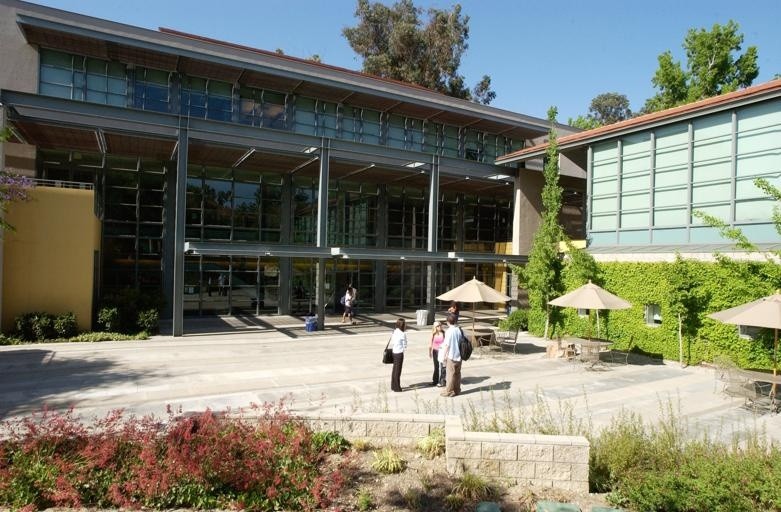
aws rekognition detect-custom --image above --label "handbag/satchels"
[382,349,394,364]
[340,295,345,305]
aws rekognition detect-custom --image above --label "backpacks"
[458,326,473,362]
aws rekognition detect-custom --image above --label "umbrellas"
[435,280,513,332]
[704,295,781,372]
[546,283,635,336]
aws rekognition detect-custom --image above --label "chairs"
[547,331,635,371]
[458,325,520,359]
[710,358,781,416]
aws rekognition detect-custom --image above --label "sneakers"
[439,390,460,397]
[428,381,444,387]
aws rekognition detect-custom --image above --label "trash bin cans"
[306,318,317,332]
[416,310,428,325]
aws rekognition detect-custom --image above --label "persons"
[208,274,213,297]
[428,321,446,388]
[217,273,224,297]
[342,280,358,326]
[391,319,409,392]
[447,302,460,324]
[441,314,466,397]
[223,275,230,297]
